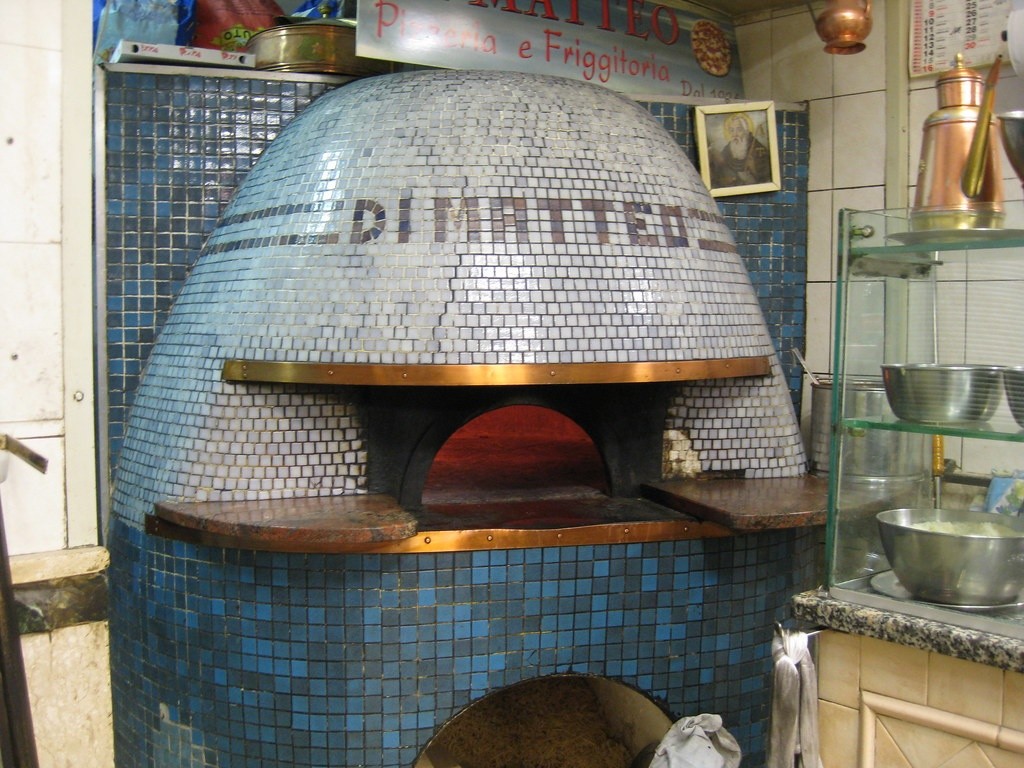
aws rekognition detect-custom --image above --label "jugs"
[910,53,1005,232]
[807,0,873,55]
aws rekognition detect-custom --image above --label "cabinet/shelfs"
[824,210,1024,639]
[813,629,1024,768]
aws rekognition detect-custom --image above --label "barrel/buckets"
[809,378,928,486]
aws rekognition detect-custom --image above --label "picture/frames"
[693,100,782,198]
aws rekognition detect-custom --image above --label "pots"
[245,7,396,76]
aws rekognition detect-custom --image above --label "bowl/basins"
[876,509,1024,615]
[880,363,1008,424]
[1002,365,1024,428]
[996,110,1024,189]
